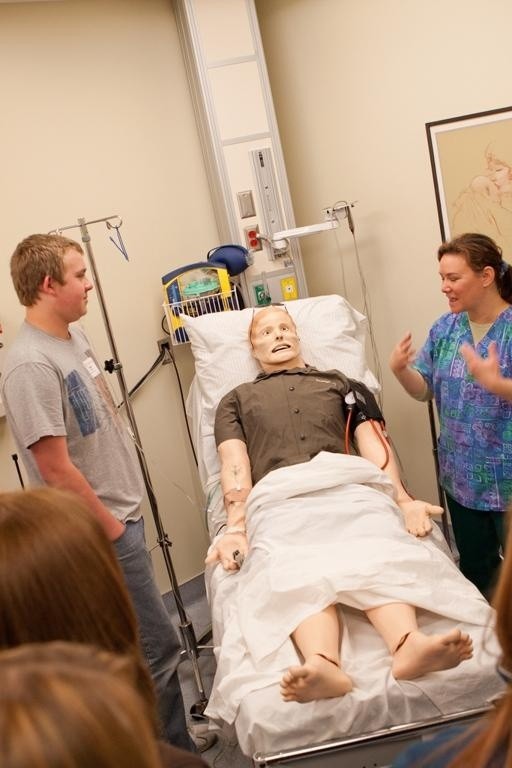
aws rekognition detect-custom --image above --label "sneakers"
[185,723,220,754]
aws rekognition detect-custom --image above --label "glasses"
[252,303,291,321]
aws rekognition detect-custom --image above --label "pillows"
[178,294,382,426]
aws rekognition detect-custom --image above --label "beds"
[183,370,511,767]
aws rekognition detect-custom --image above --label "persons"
[484,134,512,211]
[0,638,160,767]
[0,232,219,756]
[469,173,502,207]
[0,483,156,710]
[392,498,511,768]
[203,303,474,704]
[389,230,512,598]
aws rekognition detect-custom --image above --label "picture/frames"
[424,105,512,265]
